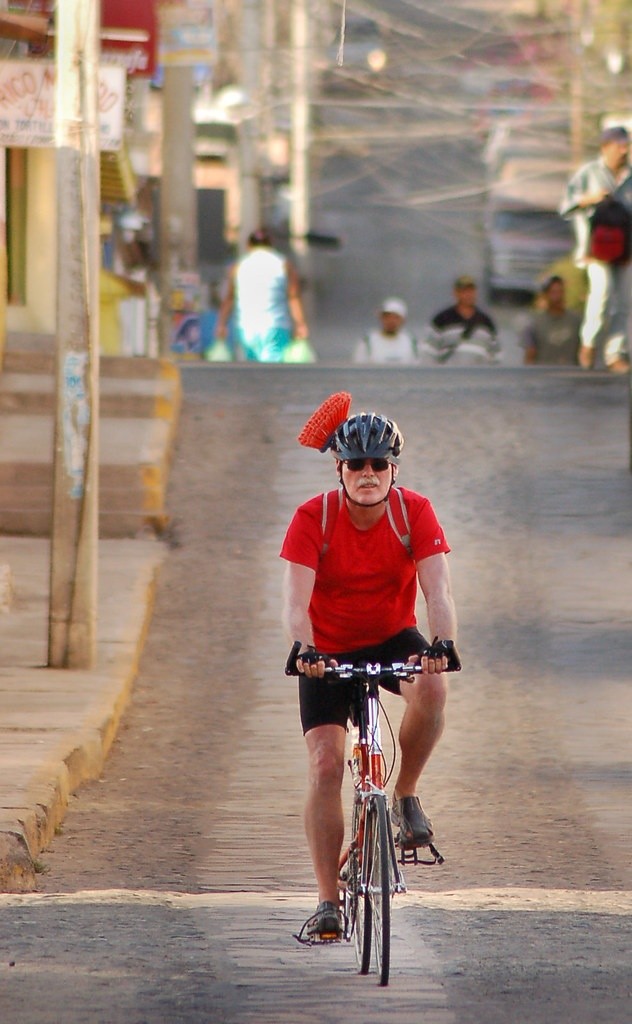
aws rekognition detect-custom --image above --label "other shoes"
[578,344,595,371]
[606,356,632,375]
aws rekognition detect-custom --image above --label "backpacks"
[588,197,632,266]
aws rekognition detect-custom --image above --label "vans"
[483,179,582,297]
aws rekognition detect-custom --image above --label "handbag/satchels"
[204,339,235,365]
[279,341,318,365]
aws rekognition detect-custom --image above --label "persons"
[416,273,503,367]
[347,295,419,365]
[215,227,310,362]
[520,275,607,371]
[558,127,632,374]
[280,412,462,935]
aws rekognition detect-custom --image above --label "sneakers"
[316,901,343,934]
[391,793,435,848]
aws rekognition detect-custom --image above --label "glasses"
[342,458,394,472]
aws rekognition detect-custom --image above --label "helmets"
[327,413,405,461]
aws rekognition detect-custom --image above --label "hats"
[382,296,409,319]
[455,276,476,290]
[598,126,626,146]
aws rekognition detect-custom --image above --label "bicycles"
[282,640,462,985]
